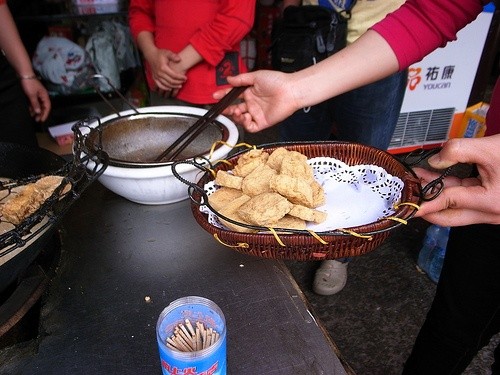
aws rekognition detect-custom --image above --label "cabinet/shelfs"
[33,68,150,156]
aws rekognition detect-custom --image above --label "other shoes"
[313,259,349,295]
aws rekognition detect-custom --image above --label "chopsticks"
[152,85,250,163]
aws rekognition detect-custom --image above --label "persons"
[129,0,255,144]
[0,0,50,159]
[212,0,500,375]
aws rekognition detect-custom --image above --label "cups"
[156,295,229,375]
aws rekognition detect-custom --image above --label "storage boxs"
[48,119,88,146]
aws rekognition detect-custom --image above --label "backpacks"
[270,0,356,112]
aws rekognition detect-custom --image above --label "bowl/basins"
[72,105,239,206]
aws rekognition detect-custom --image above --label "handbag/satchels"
[32,18,143,94]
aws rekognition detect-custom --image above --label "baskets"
[170,140,452,261]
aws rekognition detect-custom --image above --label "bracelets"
[19,75,38,80]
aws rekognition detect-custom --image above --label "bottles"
[417,222,451,283]
